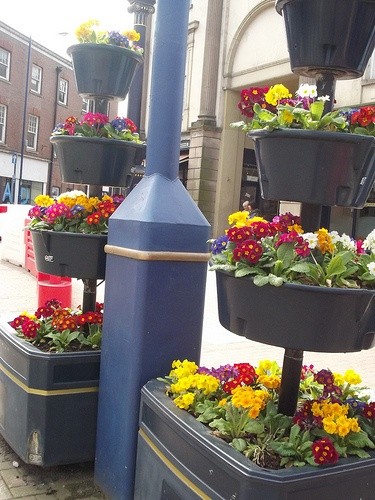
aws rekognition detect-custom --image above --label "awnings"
[131,151,189,176]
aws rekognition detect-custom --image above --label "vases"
[248,130,375,209]
[134,376,375,500]
[0,317,102,467]
[30,230,108,280]
[274,0,375,80]
[50,136,146,187]
[215,270,375,352]
[66,44,143,100]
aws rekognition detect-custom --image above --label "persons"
[242,201,251,212]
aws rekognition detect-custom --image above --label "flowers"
[75,19,144,55]
[158,356,375,469]
[206,210,375,289]
[51,112,141,140]
[23,190,127,235]
[229,83,375,138]
[8,298,107,353]
